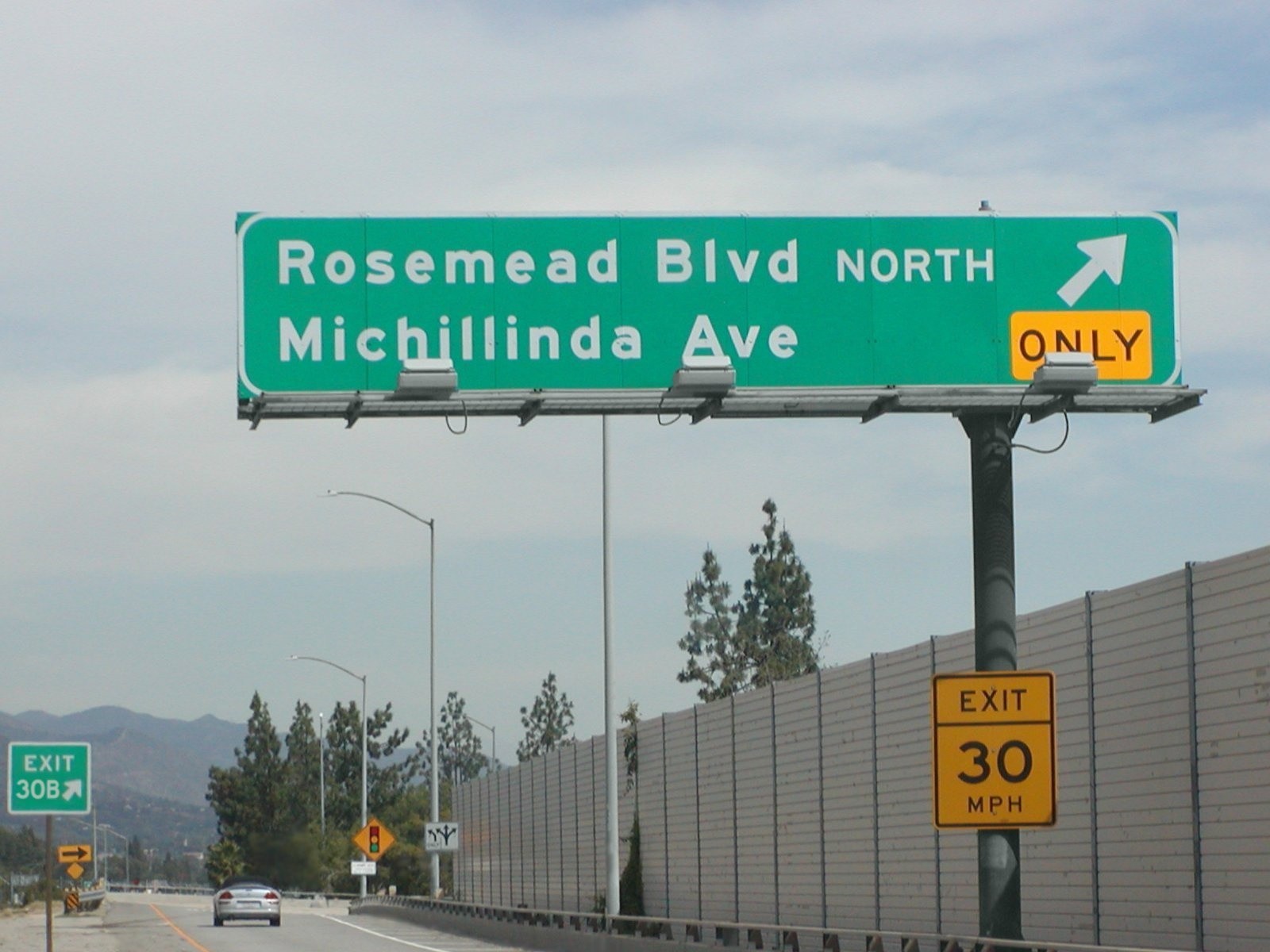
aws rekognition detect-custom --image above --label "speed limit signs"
[928,670,1058,829]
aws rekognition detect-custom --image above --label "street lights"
[449,711,496,772]
[286,654,369,897]
[97,822,112,891]
[319,490,442,900]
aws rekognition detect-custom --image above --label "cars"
[210,881,284,928]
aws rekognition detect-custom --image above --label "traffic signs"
[232,207,1187,408]
[7,740,91,815]
